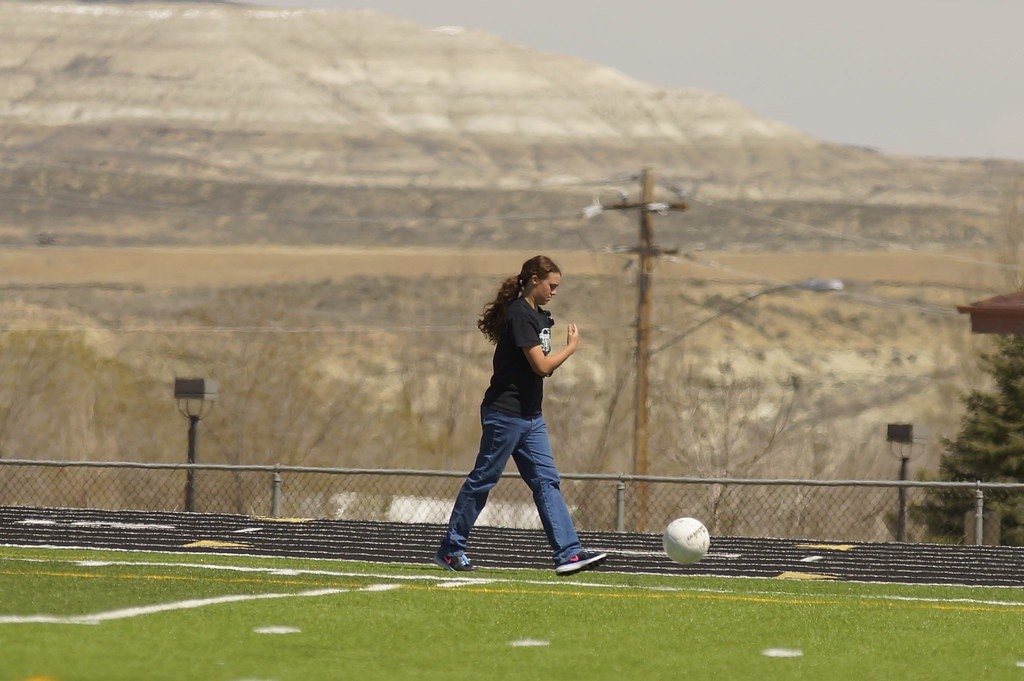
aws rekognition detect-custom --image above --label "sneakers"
[555,551,608,575]
[433,554,477,572]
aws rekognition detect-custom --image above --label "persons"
[434,255,608,575]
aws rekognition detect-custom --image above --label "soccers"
[662,517,711,564]
